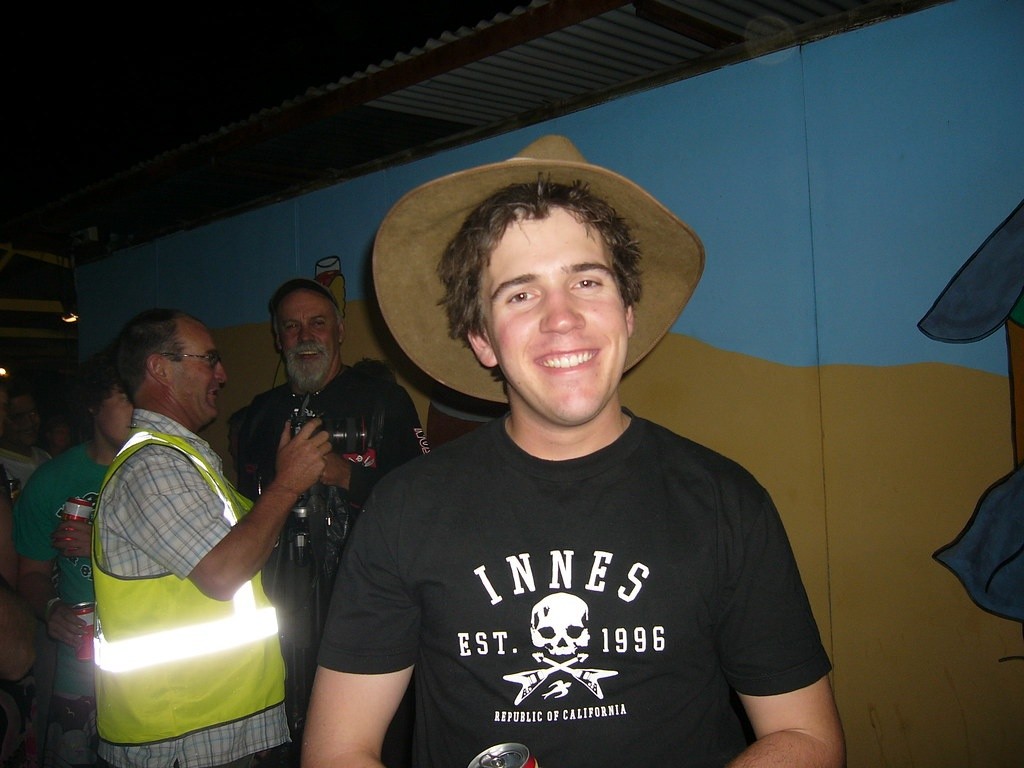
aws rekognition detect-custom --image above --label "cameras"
[290,391,369,463]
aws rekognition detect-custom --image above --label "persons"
[91,313,331,768]
[304,136,839,768]
[0,368,135,768]
[226,279,433,768]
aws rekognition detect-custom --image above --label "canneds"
[70,601,96,661]
[467,742,541,768]
[53,498,92,550]
[8,478,21,506]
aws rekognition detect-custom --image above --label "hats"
[372,135,707,403]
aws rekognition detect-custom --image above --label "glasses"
[158,352,221,369]
